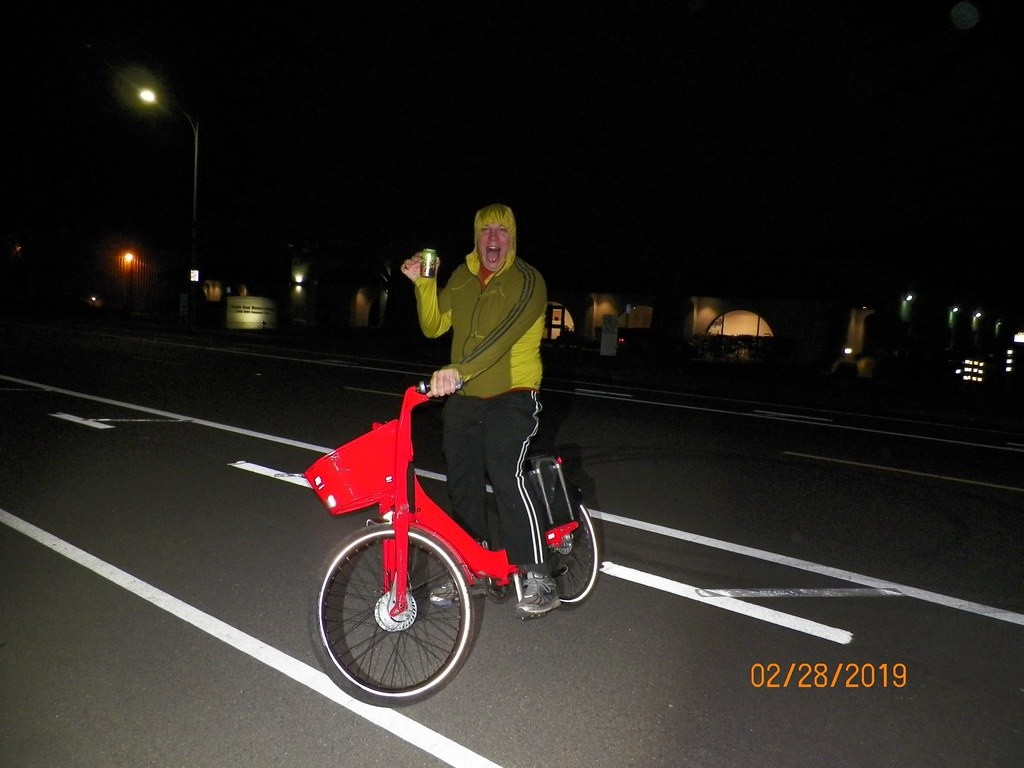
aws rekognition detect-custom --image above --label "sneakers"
[427,579,489,606]
[513,577,565,619]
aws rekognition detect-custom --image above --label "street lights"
[134,86,202,209]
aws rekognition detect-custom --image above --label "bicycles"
[303,376,602,709]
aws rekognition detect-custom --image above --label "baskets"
[305,418,400,515]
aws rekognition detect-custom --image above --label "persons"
[400,203,562,618]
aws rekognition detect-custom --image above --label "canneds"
[420,248,435,278]
